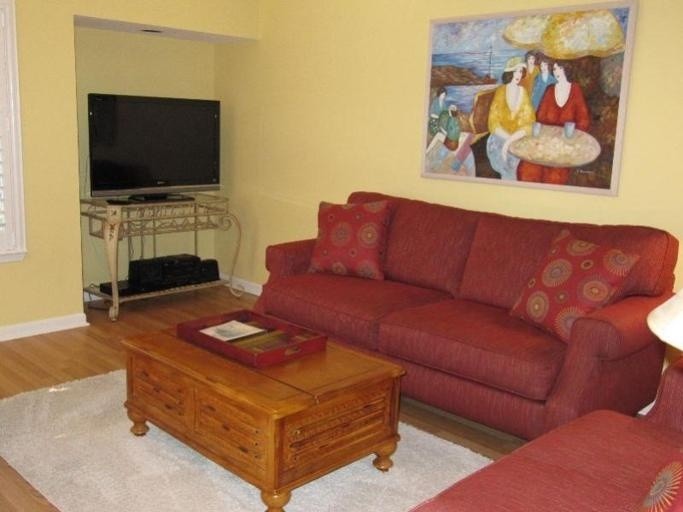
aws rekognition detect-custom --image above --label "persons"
[483,50,591,188]
[428,85,446,136]
[424,103,460,157]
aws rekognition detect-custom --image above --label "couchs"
[409,354,683,512]
[254,190,679,442]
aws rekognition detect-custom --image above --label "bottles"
[444,109,462,151]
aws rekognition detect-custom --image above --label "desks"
[639,289,683,417]
[79,192,244,321]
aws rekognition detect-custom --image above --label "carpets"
[0,365,493,512]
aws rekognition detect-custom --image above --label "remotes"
[106,199,131,205]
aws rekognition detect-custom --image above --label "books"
[198,320,264,342]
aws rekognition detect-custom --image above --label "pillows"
[509,229,640,345]
[308,199,398,282]
[633,458,683,512]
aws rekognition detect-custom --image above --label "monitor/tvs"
[86,92,221,203]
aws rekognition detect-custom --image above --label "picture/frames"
[418,0,638,199]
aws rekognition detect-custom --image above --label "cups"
[532,121,542,138]
[564,121,576,138]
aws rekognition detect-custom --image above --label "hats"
[502,55,527,74]
